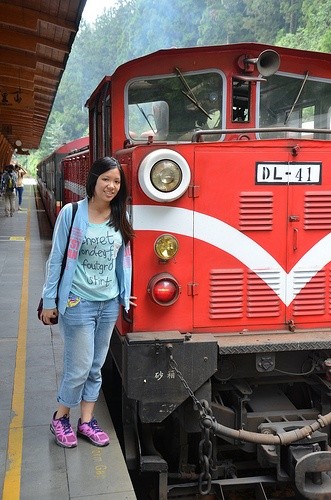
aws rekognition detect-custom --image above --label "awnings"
[0,0,87,150]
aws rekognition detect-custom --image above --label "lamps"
[15,137,22,147]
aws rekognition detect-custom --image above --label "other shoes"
[10,212,14,217]
[18,207,23,211]
[5,212,9,217]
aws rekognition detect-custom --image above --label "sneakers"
[50,410,78,448]
[76,416,109,447]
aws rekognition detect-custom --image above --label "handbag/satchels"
[37,298,59,325]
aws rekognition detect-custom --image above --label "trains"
[35,41,331,496]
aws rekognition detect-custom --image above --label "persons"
[40,156,137,449]
[0,163,27,217]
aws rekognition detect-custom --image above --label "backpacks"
[2,172,15,192]
